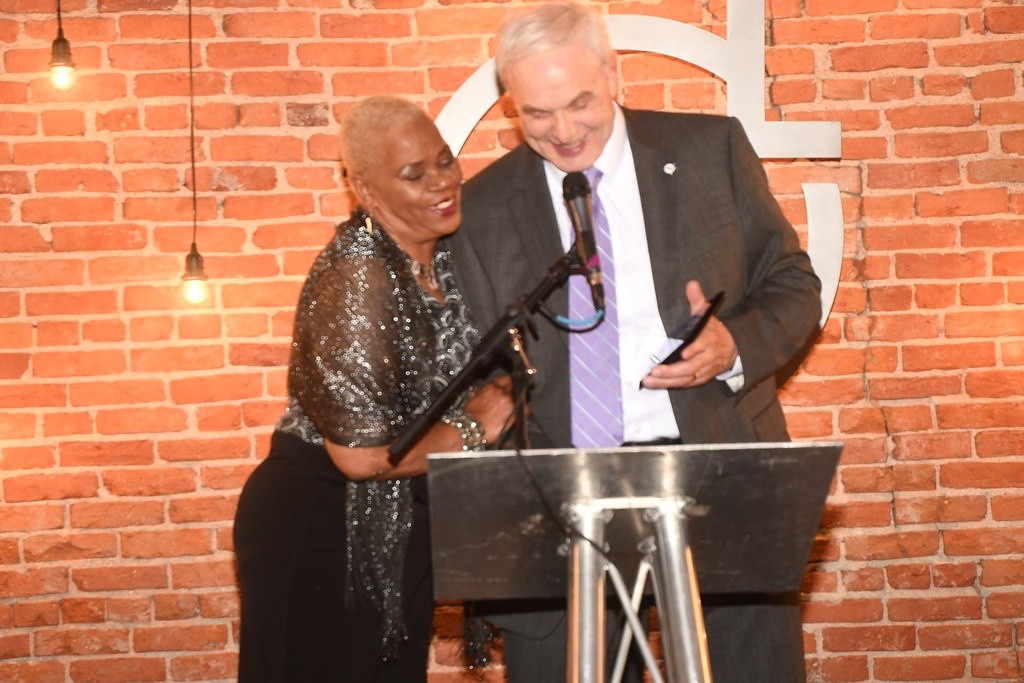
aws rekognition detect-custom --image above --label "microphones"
[564,172,606,311]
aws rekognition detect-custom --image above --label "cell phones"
[638,292,724,389]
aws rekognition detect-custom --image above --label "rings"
[693,374,697,381]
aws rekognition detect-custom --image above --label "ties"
[568,167,623,449]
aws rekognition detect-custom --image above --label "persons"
[233,95,529,683]
[437,4,822,683]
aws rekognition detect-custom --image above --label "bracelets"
[441,409,486,450]
